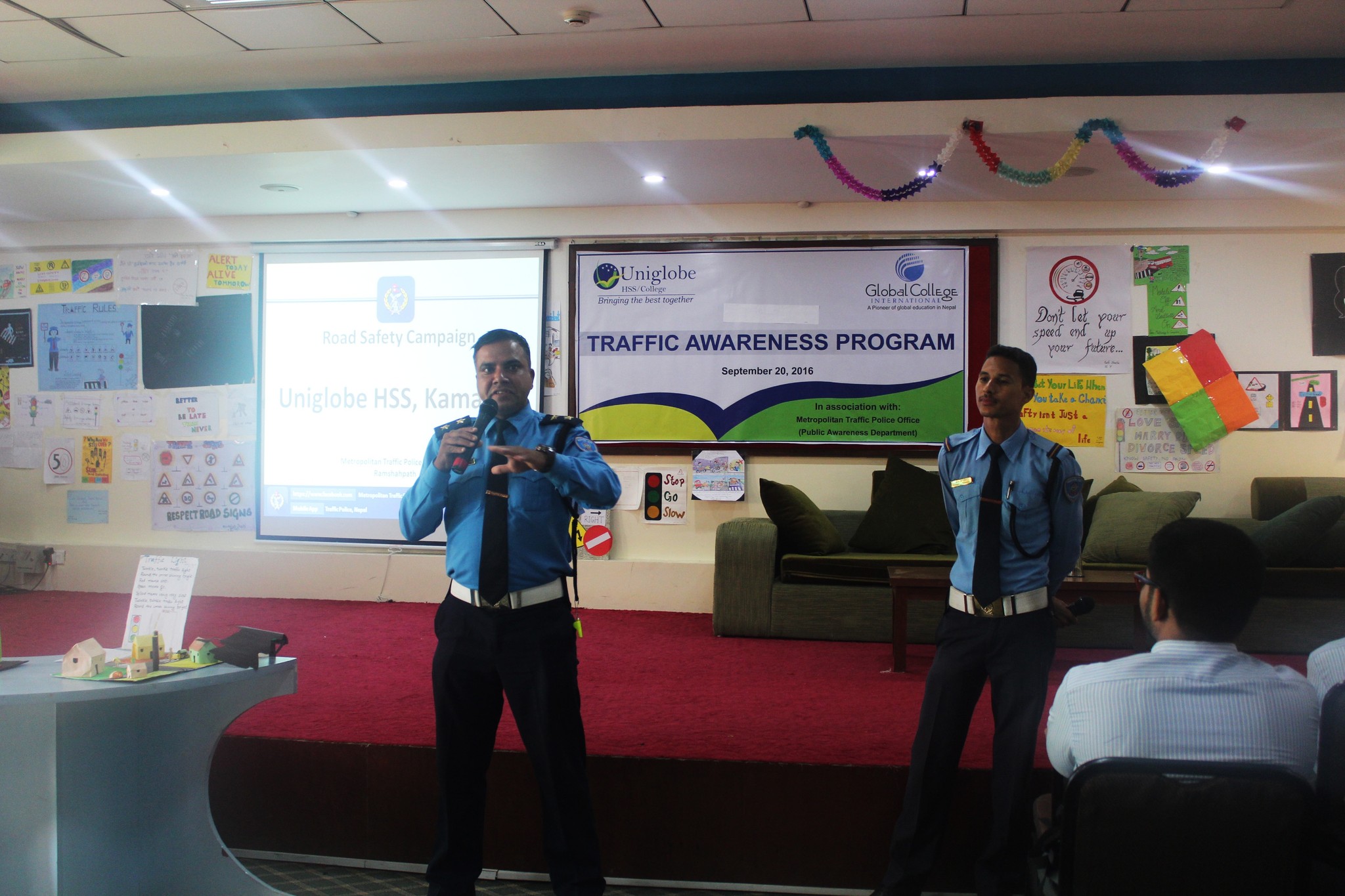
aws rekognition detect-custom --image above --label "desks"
[0,655,297,896]
[886,565,1148,672]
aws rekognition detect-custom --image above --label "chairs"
[1060,757,1315,896]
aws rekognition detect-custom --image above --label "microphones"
[451,398,498,475]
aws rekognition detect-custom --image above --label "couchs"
[712,478,1345,653]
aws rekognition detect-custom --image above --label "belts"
[948,586,1048,618]
[450,578,564,609]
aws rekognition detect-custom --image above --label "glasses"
[1134,570,1168,600]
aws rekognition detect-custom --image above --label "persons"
[1306,636,1345,776]
[869,344,1086,896]
[1045,518,1321,838]
[398,329,621,896]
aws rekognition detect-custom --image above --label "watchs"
[535,443,555,474]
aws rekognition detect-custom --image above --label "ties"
[477,419,511,607]
[972,443,1006,608]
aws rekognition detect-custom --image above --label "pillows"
[1080,475,1144,549]
[760,477,847,556]
[848,452,958,555]
[1081,491,1202,564]
[1249,493,1345,567]
